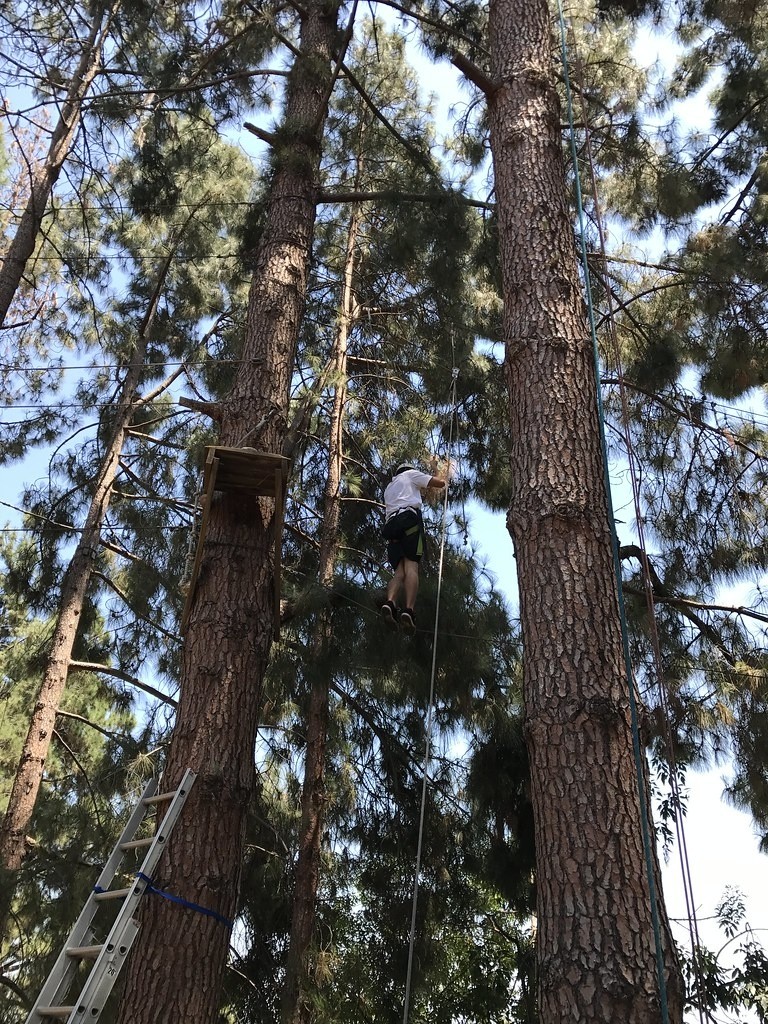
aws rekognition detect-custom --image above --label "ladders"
[24,767,198,1024]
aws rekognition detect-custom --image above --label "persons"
[381,460,446,637]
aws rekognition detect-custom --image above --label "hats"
[395,463,414,476]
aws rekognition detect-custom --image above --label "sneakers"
[401,609,416,636]
[381,602,399,632]
